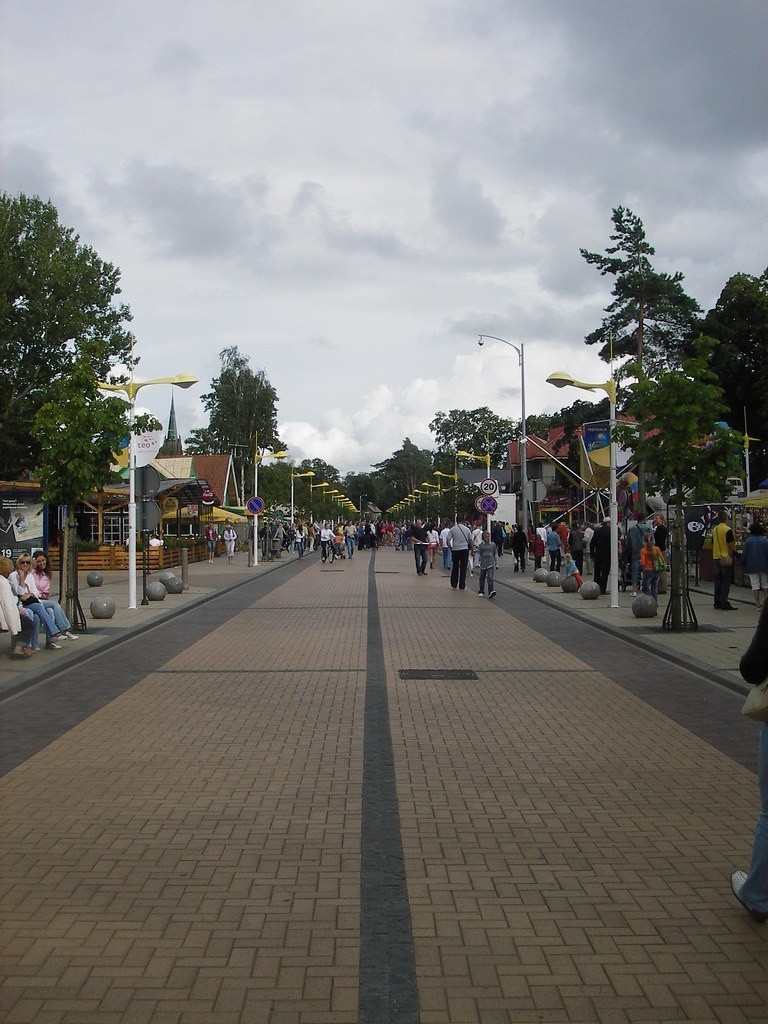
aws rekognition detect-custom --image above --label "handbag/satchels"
[582,542,586,548]
[653,555,664,572]
[742,679,768,721]
[17,594,39,607]
[501,528,507,538]
[721,556,733,566]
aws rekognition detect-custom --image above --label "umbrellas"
[737,477,768,508]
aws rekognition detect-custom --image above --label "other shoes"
[12,649,33,659]
[714,605,738,610]
[45,643,61,649]
[32,646,41,651]
[478,593,484,597]
[63,632,79,641]
[489,591,496,598]
[418,572,427,576]
[52,635,68,640]
[630,592,637,597]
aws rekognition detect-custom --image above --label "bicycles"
[321,536,336,563]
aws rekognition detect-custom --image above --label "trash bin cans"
[271,539,282,559]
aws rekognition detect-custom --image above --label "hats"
[600,517,610,525]
[225,524,233,530]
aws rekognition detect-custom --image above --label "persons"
[31,551,79,648]
[491,520,517,557]
[526,517,611,595]
[8,553,68,650]
[712,512,738,610]
[511,525,528,572]
[446,517,475,590]
[739,524,768,611]
[619,512,671,606]
[0,556,35,660]
[730,595,768,924]
[223,522,239,563]
[462,519,487,577]
[206,524,218,563]
[248,521,260,556]
[475,531,500,598]
[259,519,453,577]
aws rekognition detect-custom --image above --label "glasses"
[19,561,31,564]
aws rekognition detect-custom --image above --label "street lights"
[360,493,368,524]
[712,407,761,500]
[254,429,289,565]
[433,470,459,526]
[384,480,443,530]
[545,371,622,610]
[308,476,360,531]
[95,374,199,608]
[454,449,493,543]
[477,332,529,538]
[291,470,316,520]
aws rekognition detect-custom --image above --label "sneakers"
[730,871,768,923]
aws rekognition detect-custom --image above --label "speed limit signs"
[480,479,497,494]
